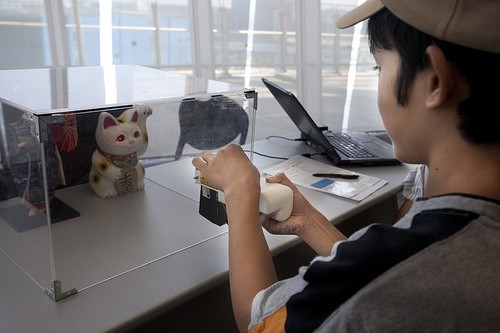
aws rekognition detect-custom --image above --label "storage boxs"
[0,62,259,302]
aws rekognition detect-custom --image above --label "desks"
[0,135,422,333]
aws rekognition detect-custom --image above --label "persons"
[192,0,500,333]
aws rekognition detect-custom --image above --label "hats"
[336,0,500,52]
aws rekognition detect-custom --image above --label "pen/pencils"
[312,173,359,180]
